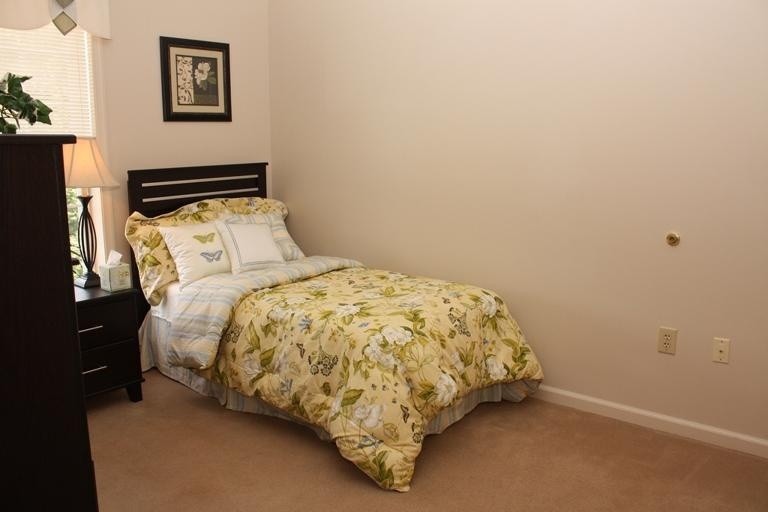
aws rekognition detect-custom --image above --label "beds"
[125,161,507,474]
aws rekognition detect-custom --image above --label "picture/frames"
[159,34,233,122]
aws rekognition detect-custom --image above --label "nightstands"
[72,279,147,403]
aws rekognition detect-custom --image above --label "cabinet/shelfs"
[2,135,104,512]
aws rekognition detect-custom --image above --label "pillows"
[123,194,308,308]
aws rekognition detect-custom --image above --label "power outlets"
[657,326,678,355]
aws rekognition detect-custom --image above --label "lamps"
[60,135,122,289]
